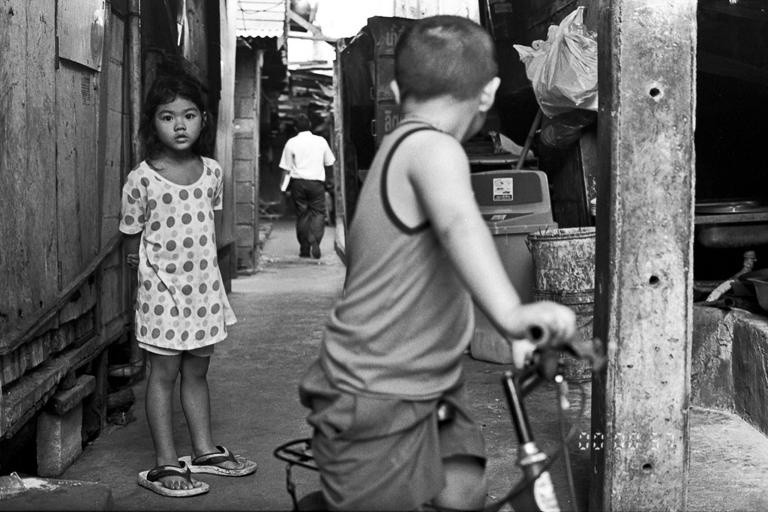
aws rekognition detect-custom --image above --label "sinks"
[695,199,768,256]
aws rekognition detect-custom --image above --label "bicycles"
[273,323,607,507]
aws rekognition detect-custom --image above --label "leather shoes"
[299,252,310,257]
[308,234,321,258]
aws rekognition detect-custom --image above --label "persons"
[298,15,575,512]
[118,73,257,498]
[279,114,336,258]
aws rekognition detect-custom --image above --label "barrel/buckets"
[524,289,595,382]
[524,225,596,294]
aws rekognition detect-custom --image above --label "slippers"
[177,446,257,477]
[137,460,209,497]
